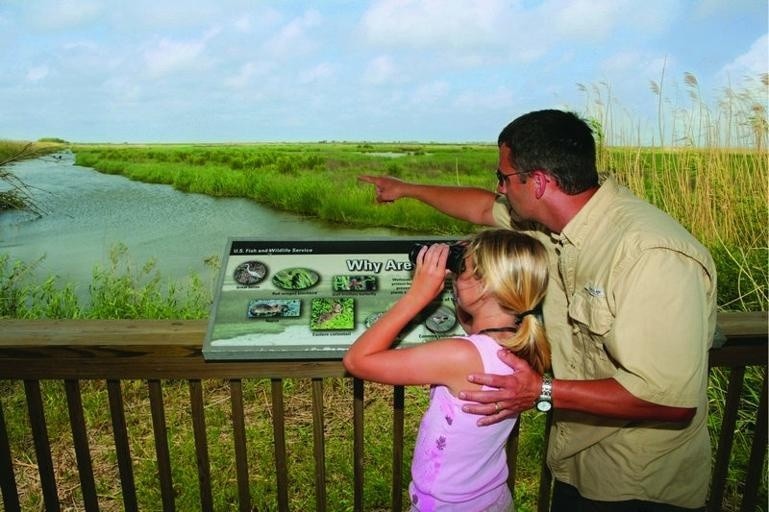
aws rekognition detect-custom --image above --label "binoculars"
[408,243,466,275]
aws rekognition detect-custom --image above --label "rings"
[495,402,500,414]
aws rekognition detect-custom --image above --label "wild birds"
[245,263,264,281]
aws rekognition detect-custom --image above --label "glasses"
[497,168,545,187]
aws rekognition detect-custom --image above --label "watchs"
[535,374,553,413]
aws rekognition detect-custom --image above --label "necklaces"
[478,326,517,334]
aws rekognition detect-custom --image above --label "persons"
[356,108,718,512]
[343,228,552,512]
